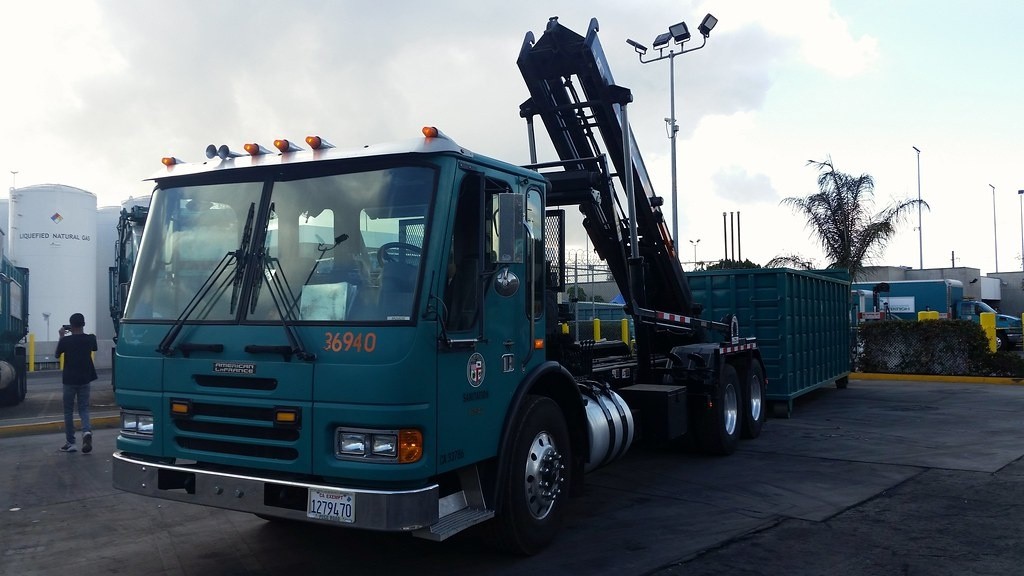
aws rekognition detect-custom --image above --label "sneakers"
[60,441,77,452]
[82,431,92,453]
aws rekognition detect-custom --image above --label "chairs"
[271,255,323,304]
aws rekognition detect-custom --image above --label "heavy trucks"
[0,254,30,408]
[109,15,769,563]
[107,202,273,392]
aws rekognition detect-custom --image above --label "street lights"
[989,183,999,274]
[626,13,719,253]
[1017,190,1024,272]
[912,145,923,268]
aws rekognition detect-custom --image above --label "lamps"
[970,278,977,283]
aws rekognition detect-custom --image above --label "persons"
[55,313,99,453]
[994,306,1002,314]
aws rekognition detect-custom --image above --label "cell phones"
[62,325,72,330]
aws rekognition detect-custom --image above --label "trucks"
[852,279,1023,352]
